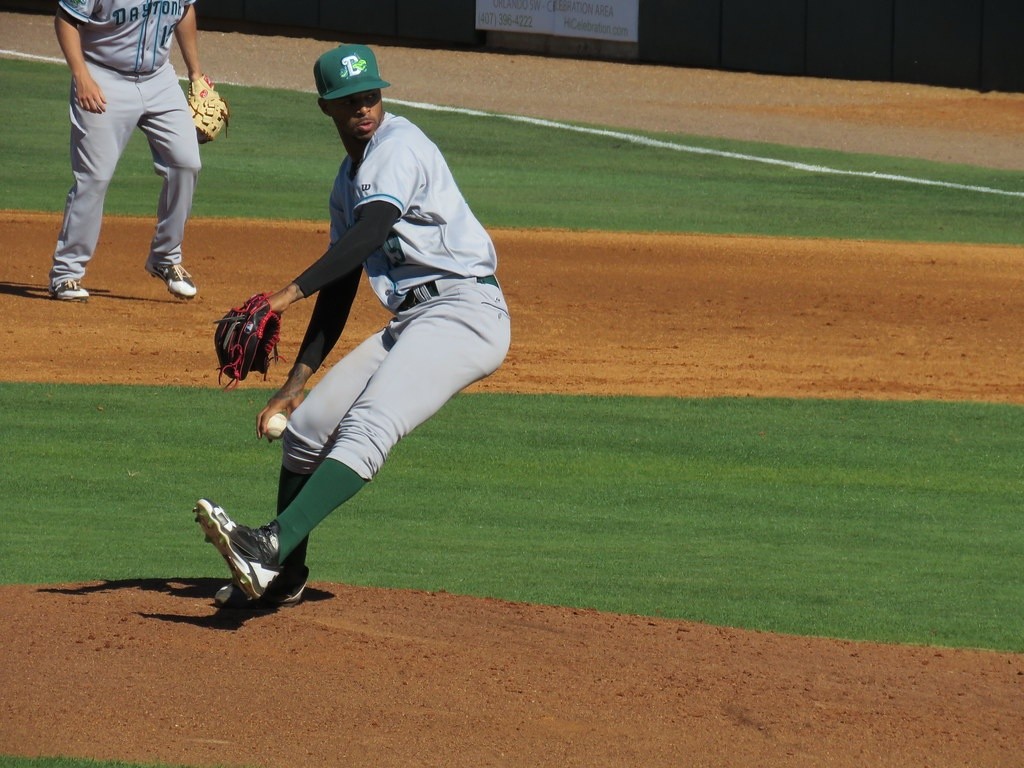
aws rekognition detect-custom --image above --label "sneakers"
[193,498,285,601]
[145,262,197,300]
[49,280,90,302]
[215,563,309,612]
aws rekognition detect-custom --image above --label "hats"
[314,44,391,100]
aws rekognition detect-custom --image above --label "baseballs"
[264,412,288,440]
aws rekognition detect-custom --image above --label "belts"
[398,275,500,312]
[83,52,164,77]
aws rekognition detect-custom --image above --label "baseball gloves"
[186,72,232,145]
[209,290,288,394]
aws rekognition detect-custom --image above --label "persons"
[49,0,226,299]
[191,44,510,604]
